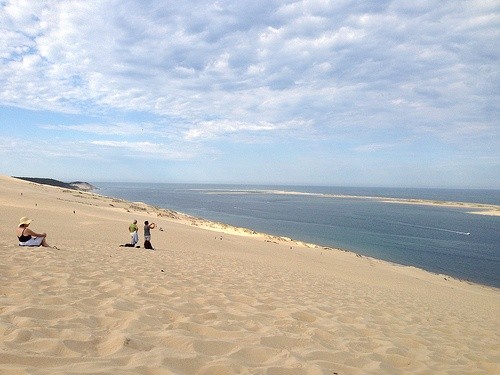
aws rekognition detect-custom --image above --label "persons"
[128,220,139,245]
[143,220,156,249]
[14,216,59,250]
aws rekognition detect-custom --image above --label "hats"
[17,216,33,228]
